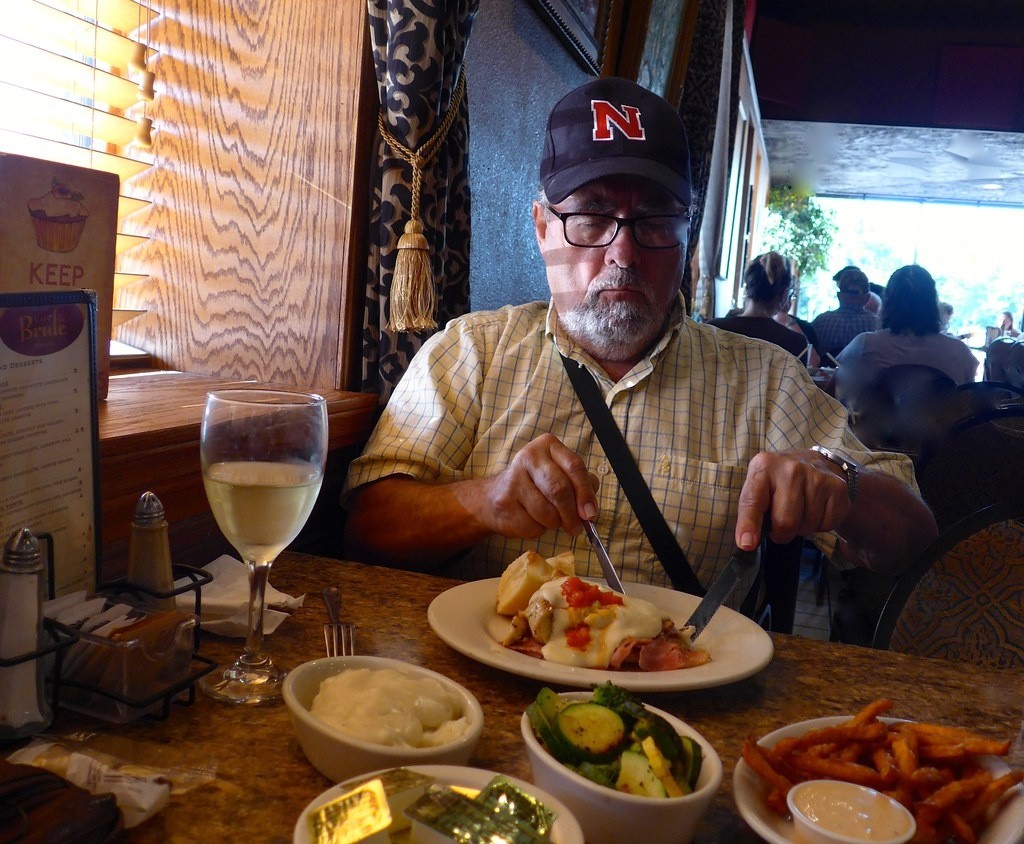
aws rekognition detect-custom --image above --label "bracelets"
[786,318,796,327]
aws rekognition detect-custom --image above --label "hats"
[838,269,869,295]
[539,77,692,208]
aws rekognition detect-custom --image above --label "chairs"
[856,363,959,455]
[913,336,1023,539]
[870,493,1023,663]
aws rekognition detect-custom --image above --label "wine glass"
[194,388,329,704]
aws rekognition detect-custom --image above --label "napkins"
[173,554,307,638]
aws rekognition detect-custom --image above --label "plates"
[292,766,584,844]
[732,717,1024,844]
[428,578,773,691]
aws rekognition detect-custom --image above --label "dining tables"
[0,549,1022,844]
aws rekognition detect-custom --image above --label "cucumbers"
[524,679,704,798]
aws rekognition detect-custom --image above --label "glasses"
[545,206,692,250]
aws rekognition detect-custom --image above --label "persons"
[725,297,819,369]
[966,312,1021,353]
[344,77,939,610]
[810,266,886,358]
[835,264,981,407]
[939,302,973,340]
[706,252,807,368]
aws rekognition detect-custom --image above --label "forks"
[321,587,355,657]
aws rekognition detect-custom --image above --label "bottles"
[0,527,52,738]
[128,492,176,610]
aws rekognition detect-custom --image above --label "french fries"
[737,696,1024,844]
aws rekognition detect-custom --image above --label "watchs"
[808,444,859,503]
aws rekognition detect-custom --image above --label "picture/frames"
[623,0,700,112]
[716,97,748,282]
[520,0,615,77]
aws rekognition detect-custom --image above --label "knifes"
[582,519,629,594]
[684,516,773,642]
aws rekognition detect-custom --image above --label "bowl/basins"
[521,692,723,844]
[786,780,916,844]
[282,657,483,785]
